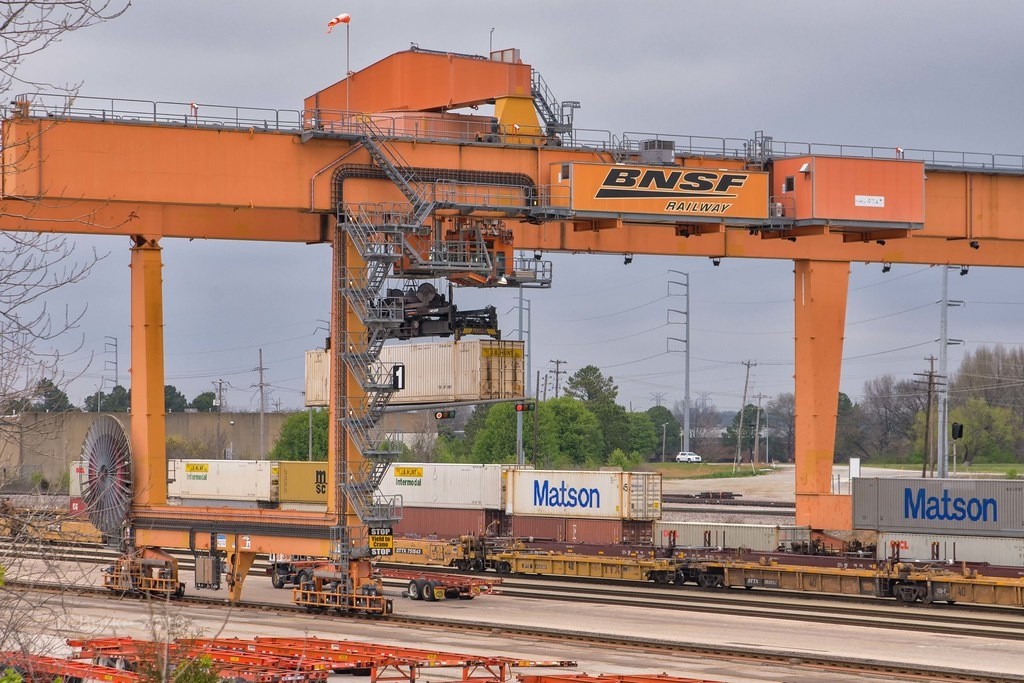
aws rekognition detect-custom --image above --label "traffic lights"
[515,402,535,411]
[435,411,455,419]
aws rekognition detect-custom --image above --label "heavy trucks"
[267,552,504,601]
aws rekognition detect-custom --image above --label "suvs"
[676,451,701,463]
